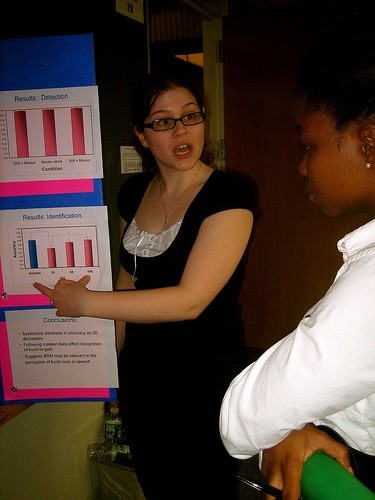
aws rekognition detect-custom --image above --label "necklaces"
[159,161,203,226]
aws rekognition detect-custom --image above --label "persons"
[218,27,375,500]
[31,67,262,499]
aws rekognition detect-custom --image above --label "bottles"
[104,400,125,444]
[86,443,133,465]
[300,450,375,500]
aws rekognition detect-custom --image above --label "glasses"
[142,112,207,132]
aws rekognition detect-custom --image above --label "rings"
[50,300,54,306]
